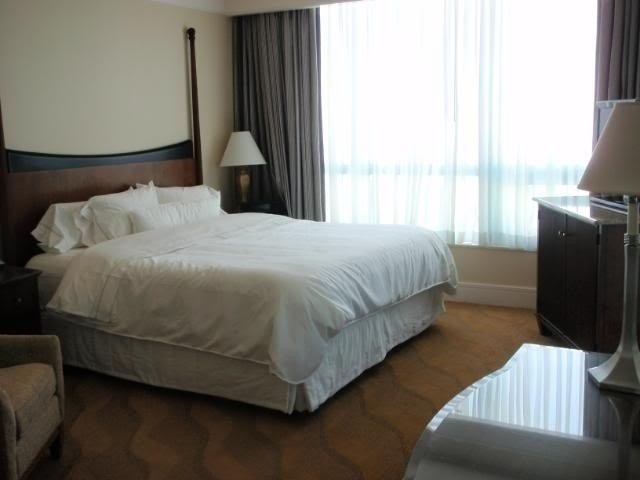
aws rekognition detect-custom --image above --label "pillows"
[82,179,221,244]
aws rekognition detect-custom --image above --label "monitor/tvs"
[588,99,640,215]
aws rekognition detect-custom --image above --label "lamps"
[219,130,268,206]
[579,100,639,396]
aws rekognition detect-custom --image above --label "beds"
[0,28,458,417]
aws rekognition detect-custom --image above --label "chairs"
[0,334,66,480]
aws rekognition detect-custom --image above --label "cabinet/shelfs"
[532,196,627,353]
